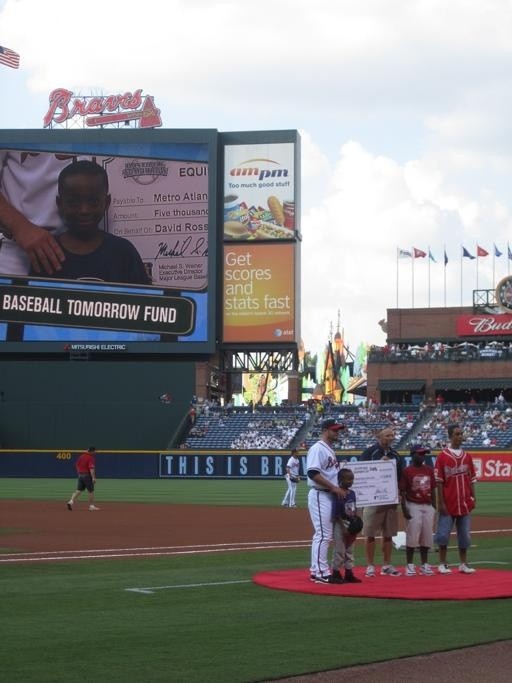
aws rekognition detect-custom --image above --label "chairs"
[183,411,510,451]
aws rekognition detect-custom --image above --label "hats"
[88,446,96,450]
[291,447,299,451]
[322,419,344,431]
[410,445,430,454]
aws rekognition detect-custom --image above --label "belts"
[313,488,328,492]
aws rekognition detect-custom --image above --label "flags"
[396,241,511,265]
[0,45,21,68]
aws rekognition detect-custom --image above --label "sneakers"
[88,506,99,510]
[310,564,476,584]
[67,502,72,510]
[281,501,297,509]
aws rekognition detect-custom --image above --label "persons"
[331,468,362,583]
[358,428,403,579]
[31,159,151,284]
[433,423,477,576]
[1,148,65,275]
[370,339,511,360]
[280,448,300,509]
[306,418,350,584]
[67,447,99,511]
[399,443,436,577]
